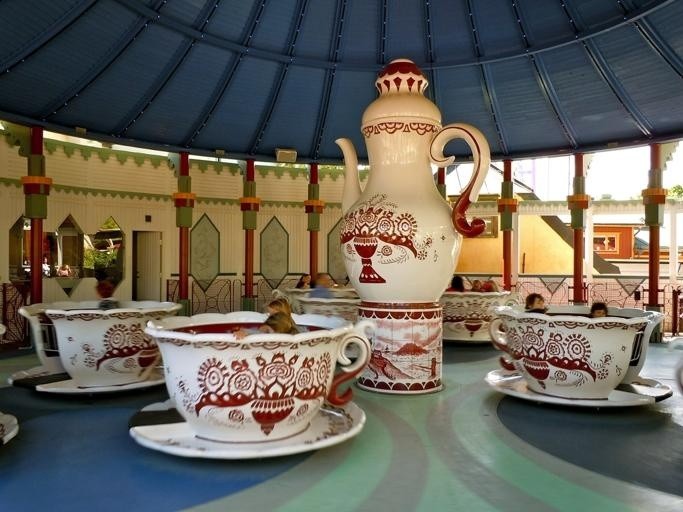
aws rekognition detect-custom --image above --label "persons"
[232,298,300,340]
[295,274,311,288]
[95,282,121,310]
[42,257,51,276]
[590,302,608,318]
[307,273,334,298]
[525,293,548,313]
[445,276,498,292]
[56,264,71,276]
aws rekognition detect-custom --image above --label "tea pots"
[336,56,492,306]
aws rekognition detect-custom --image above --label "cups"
[444,290,512,337]
[144,311,371,443]
[18,298,182,386]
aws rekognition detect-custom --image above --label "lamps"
[274,148,299,163]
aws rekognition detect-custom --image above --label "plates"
[7,366,165,394]
[131,391,368,463]
[482,368,674,410]
[443,338,492,343]
